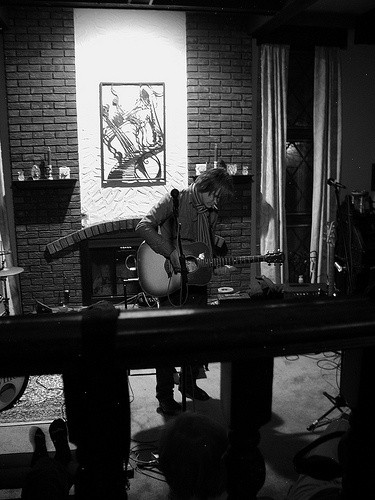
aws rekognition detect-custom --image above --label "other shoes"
[156,390,182,413]
[179,377,209,401]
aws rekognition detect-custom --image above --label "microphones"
[326,178,347,190]
[171,188,180,211]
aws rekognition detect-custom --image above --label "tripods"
[305,188,350,430]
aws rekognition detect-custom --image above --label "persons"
[136,168,232,414]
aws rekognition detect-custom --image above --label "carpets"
[0,374,68,426]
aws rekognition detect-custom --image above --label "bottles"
[31,165,40,180]
[298,275,304,283]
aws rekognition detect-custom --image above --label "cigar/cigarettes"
[213,205,218,209]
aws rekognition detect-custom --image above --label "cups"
[227,163,237,176]
[59,166,71,180]
[242,165,249,175]
[18,171,24,181]
[195,164,207,176]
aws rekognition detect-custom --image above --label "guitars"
[137,237,286,298]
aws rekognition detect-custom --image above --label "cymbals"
[0,266,24,276]
